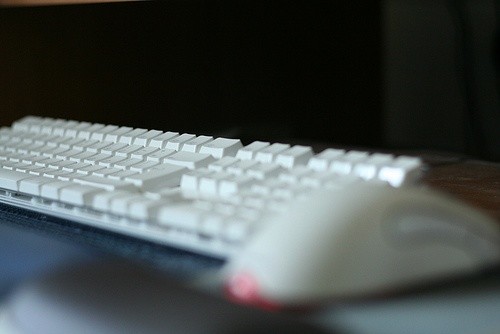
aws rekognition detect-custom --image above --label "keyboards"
[0,114,425,264]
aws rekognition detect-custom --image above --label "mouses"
[224,179,496,312]
